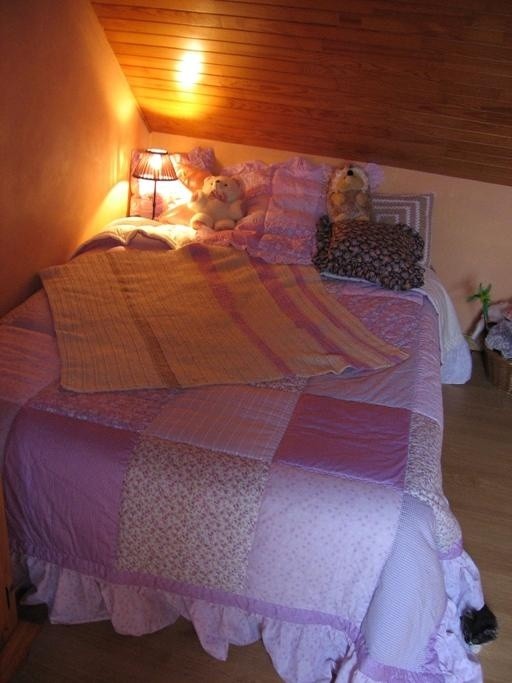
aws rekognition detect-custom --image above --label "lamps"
[133,150,179,221]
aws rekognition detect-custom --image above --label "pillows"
[313,215,425,290]
[371,191,436,268]
[197,158,383,266]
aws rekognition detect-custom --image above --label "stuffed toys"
[189,175,248,231]
[328,163,372,222]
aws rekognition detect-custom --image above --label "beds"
[1,165,489,682]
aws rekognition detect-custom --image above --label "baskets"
[484,340,512,393]
